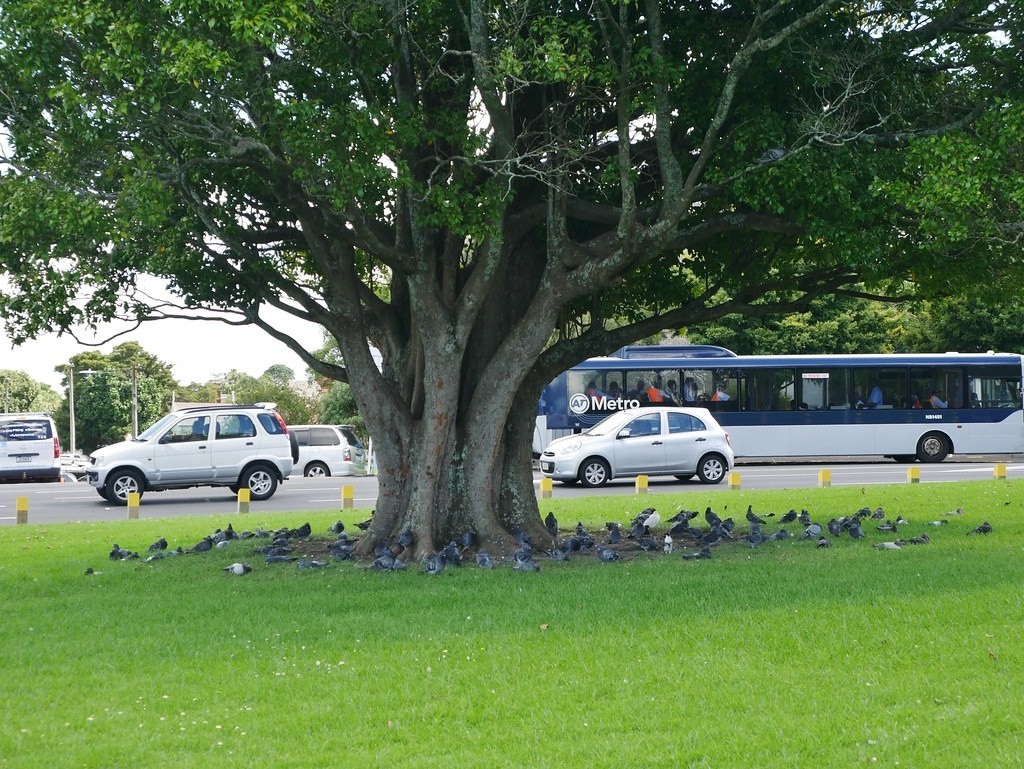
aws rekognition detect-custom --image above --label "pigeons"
[84,500,993,577]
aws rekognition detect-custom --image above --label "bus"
[543,345,1023,463]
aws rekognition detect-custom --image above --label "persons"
[858,378,951,409]
[189,421,206,441]
[583,376,731,402]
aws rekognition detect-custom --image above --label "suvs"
[87,402,300,508]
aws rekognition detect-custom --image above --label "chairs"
[205,422,221,438]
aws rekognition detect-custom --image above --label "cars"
[539,406,734,489]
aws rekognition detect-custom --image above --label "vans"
[241,424,367,479]
[0,414,62,482]
